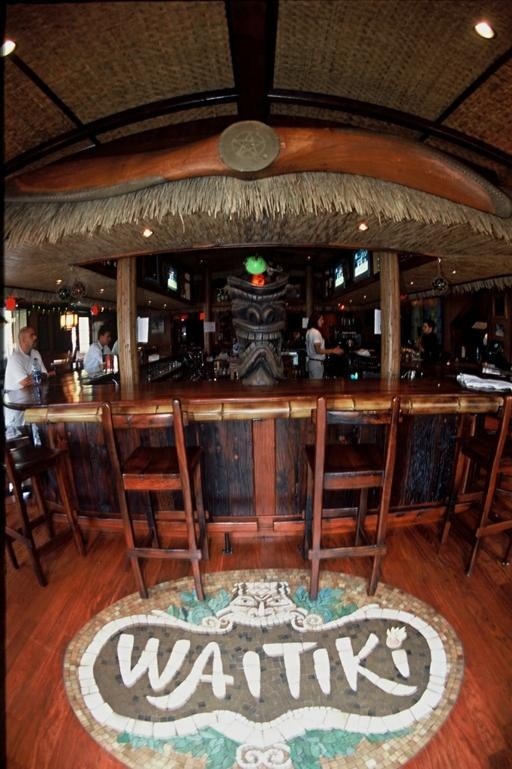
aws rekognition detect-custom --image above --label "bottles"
[460,344,465,359]
[32,386,43,406]
[148,358,182,382]
[32,357,42,385]
[102,349,114,373]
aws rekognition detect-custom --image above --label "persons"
[418,318,441,379]
[111,335,118,356]
[1,326,51,497]
[303,310,344,381]
[83,324,112,374]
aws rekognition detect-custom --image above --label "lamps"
[60,304,78,331]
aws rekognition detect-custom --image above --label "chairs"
[440,395,512,576]
[101,398,210,601]
[3,424,88,587]
[303,396,401,601]
